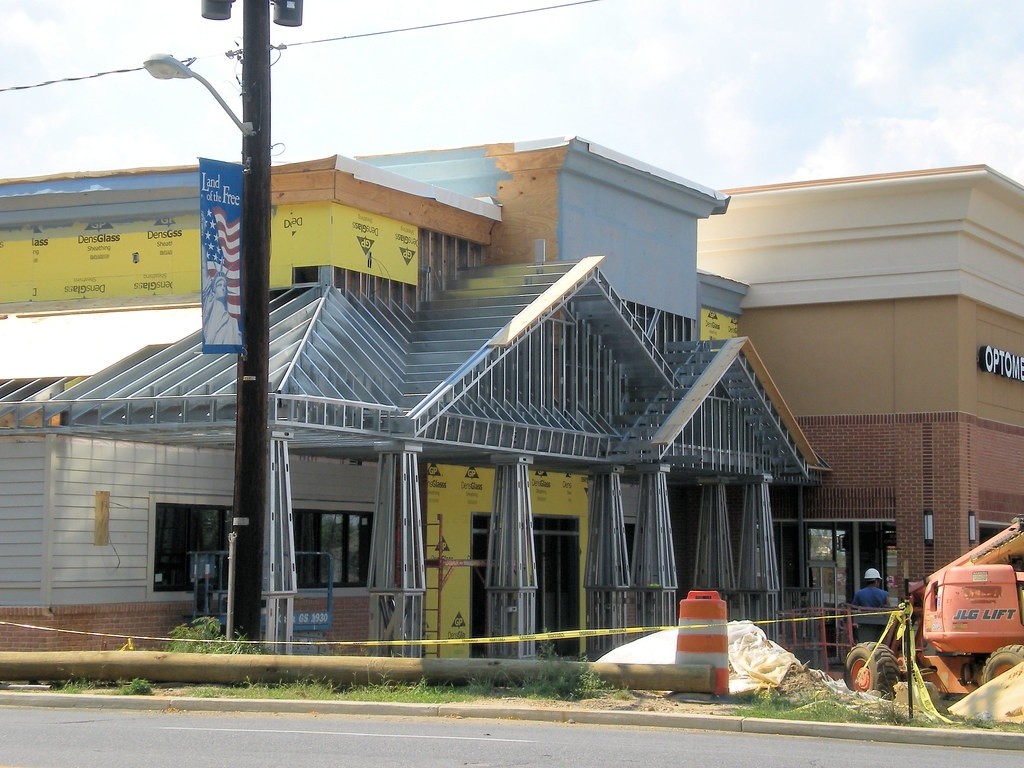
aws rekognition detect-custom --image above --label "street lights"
[145,53,271,650]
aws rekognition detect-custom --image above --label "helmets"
[864,568,882,579]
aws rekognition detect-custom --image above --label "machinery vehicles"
[843,516,1024,698]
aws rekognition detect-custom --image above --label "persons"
[852,569,890,645]
[201,213,242,344]
